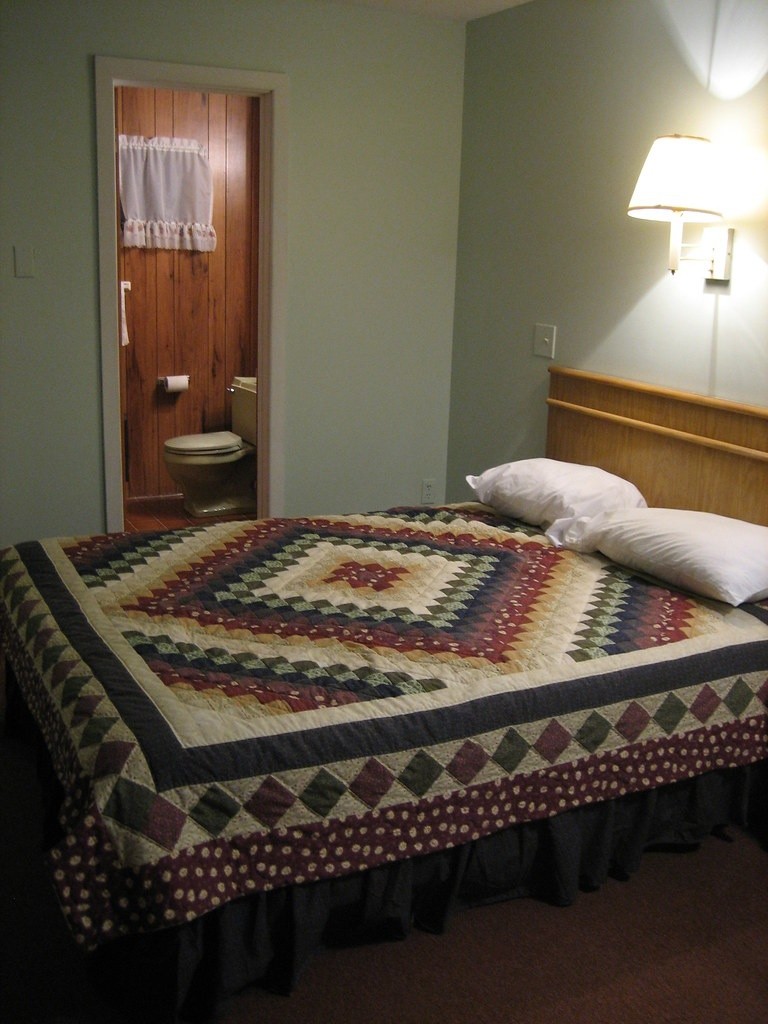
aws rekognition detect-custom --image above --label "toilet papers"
[164,375,189,393]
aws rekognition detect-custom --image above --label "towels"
[121,280,130,347]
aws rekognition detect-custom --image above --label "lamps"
[626,134,735,280]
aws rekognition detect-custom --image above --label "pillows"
[465,457,648,554]
[582,507,768,607]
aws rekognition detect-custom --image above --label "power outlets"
[422,479,435,504]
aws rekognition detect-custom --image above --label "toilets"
[163,376,257,518]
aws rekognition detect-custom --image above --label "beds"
[0,364,768,1024]
